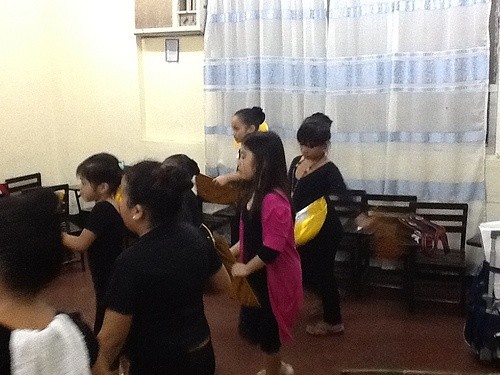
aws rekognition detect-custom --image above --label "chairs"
[331,189,469,317]
[5,173,86,273]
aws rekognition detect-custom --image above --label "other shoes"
[257,361,294,375]
[305,321,344,335]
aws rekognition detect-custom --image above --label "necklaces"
[291,152,324,198]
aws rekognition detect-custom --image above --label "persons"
[0,152,232,375]
[211,106,265,191]
[229,130,304,375]
[287,112,346,335]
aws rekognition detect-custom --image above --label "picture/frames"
[165,39,180,62]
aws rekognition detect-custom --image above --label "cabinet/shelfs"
[133,0,208,38]
